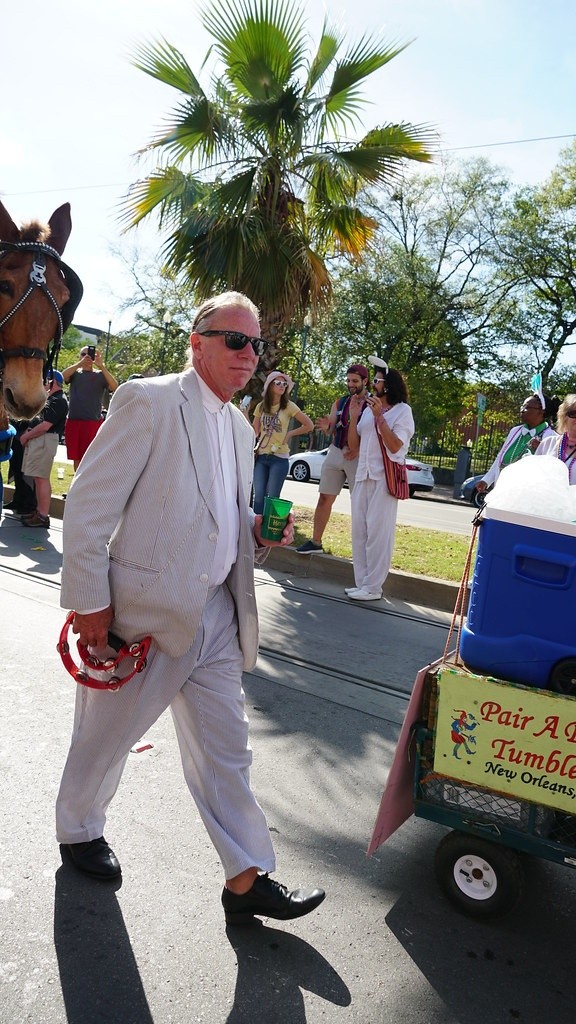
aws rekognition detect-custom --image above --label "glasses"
[373,378,385,385]
[199,330,270,357]
[566,410,576,418]
[519,406,540,411]
[272,379,288,387]
[81,352,86,356]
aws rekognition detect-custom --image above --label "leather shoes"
[60,835,122,879]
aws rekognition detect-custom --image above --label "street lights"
[286,310,314,445]
[102,317,113,364]
[160,310,172,377]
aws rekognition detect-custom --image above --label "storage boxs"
[457,506,576,694]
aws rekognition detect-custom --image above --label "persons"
[62,346,118,473]
[296,364,374,554]
[60,290,327,920]
[2,369,68,527]
[475,394,559,492]
[344,368,415,599]
[243,371,314,515]
[534,394,576,485]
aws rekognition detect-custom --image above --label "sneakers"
[295,540,323,554]
[345,587,361,594]
[348,590,381,601]
[21,511,50,528]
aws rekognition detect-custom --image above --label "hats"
[262,372,293,394]
[46,368,63,384]
[348,364,370,386]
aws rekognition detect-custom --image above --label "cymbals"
[56,610,149,694]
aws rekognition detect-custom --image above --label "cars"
[460,470,495,509]
[285,447,435,497]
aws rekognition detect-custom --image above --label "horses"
[0,201,73,423]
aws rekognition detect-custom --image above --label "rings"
[372,403,375,407]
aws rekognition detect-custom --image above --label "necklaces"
[499,425,549,465]
[558,432,576,482]
[258,408,280,448]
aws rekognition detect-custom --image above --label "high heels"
[221,873,326,926]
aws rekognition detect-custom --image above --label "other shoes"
[17,505,34,514]
[3,500,18,509]
[63,493,67,498]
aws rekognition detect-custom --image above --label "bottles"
[239,395,252,411]
[466,439,472,447]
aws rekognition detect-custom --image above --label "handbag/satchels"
[384,459,409,500]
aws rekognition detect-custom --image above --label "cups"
[261,495,293,542]
[57,468,65,479]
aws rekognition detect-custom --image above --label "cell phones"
[88,345,95,361]
